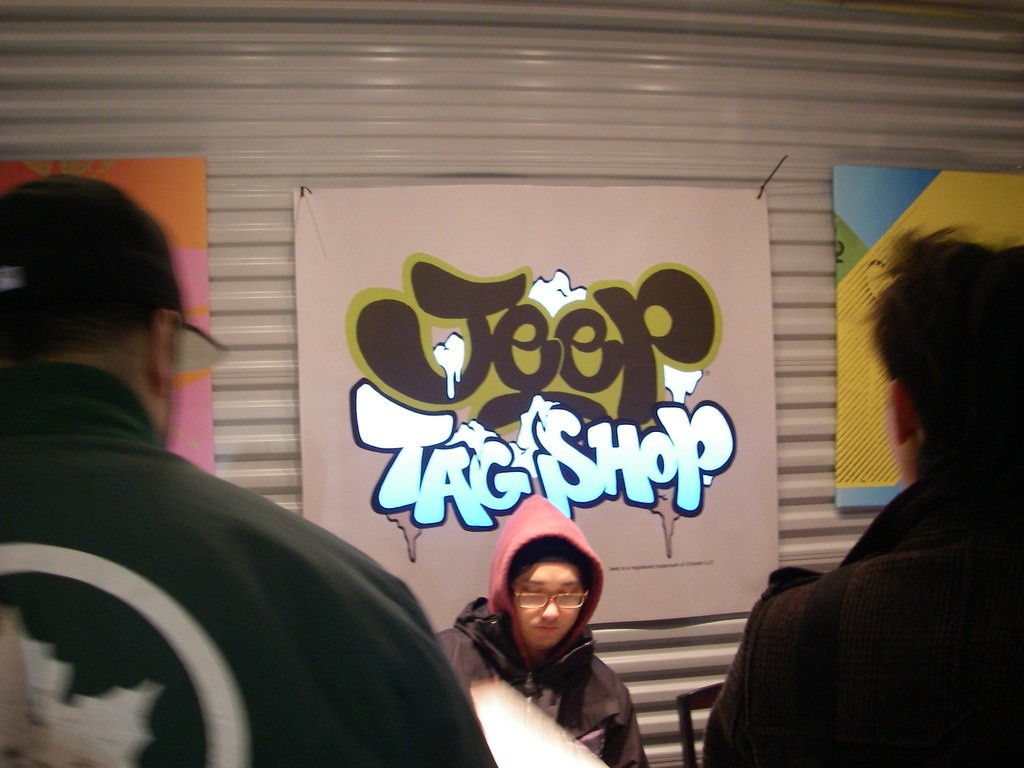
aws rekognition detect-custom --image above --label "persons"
[699,223,1023,768]
[433,493,648,767]
[0,174,501,768]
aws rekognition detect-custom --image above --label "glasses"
[510,591,590,608]
[174,319,229,373]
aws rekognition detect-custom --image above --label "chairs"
[677,681,724,768]
